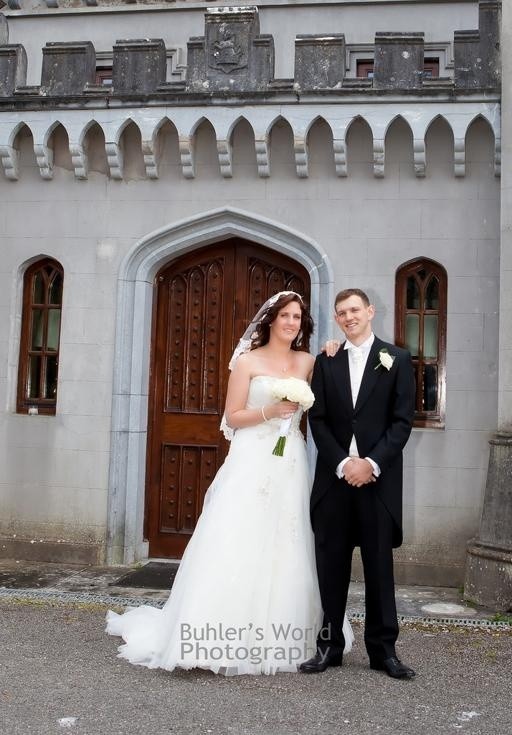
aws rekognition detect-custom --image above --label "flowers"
[271,376,316,456]
[374,347,396,372]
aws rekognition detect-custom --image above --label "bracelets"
[259,405,271,424]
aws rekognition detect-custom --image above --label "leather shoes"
[298,646,342,672]
[371,656,415,679]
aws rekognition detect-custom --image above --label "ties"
[349,349,362,407]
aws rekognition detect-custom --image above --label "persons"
[300,288,415,678]
[105,289,356,674]
